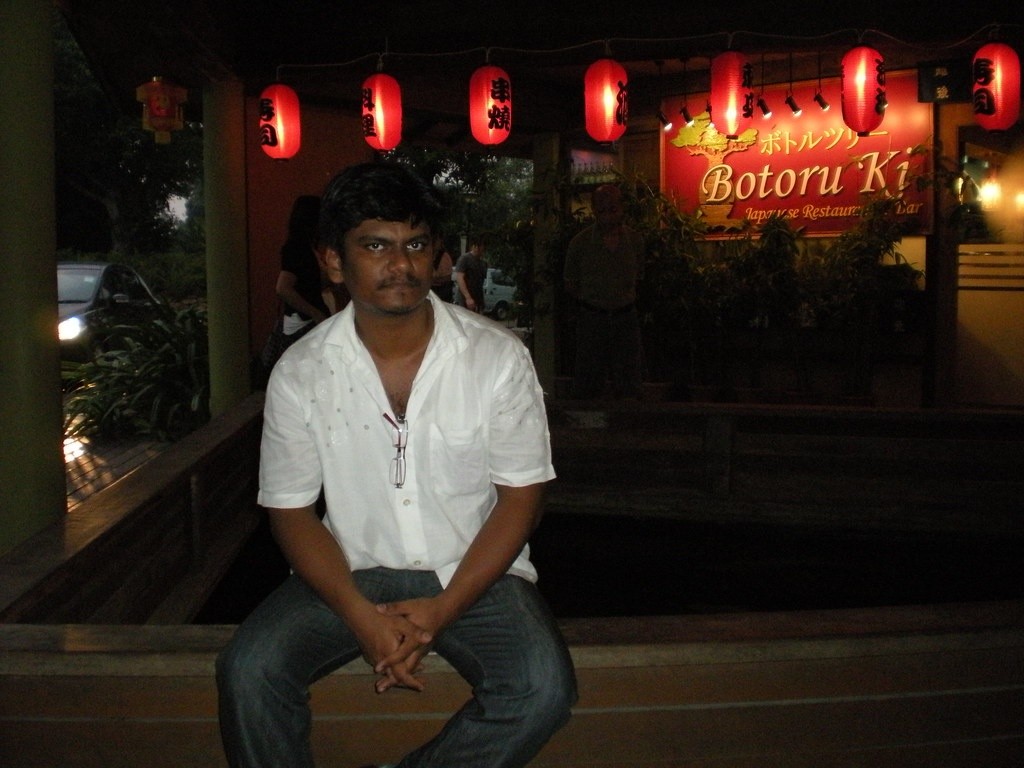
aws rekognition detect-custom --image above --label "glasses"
[382,413,409,488]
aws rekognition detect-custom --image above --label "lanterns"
[712,50,754,140]
[361,75,403,155]
[840,47,886,137]
[136,76,188,143]
[584,59,628,146]
[973,42,1022,133]
[259,84,304,161]
[470,65,512,148]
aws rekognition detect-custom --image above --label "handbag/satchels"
[259,319,312,367]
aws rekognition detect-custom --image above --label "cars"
[58,262,168,388]
[480,270,523,319]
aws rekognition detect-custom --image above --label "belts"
[580,300,633,317]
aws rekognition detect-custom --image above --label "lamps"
[679,57,695,126]
[755,48,773,120]
[814,40,831,111]
[784,43,802,116]
[654,60,672,132]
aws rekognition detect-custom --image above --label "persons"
[214,158,577,768]
[454,241,489,314]
[431,238,453,304]
[263,196,344,370]
[564,183,647,386]
